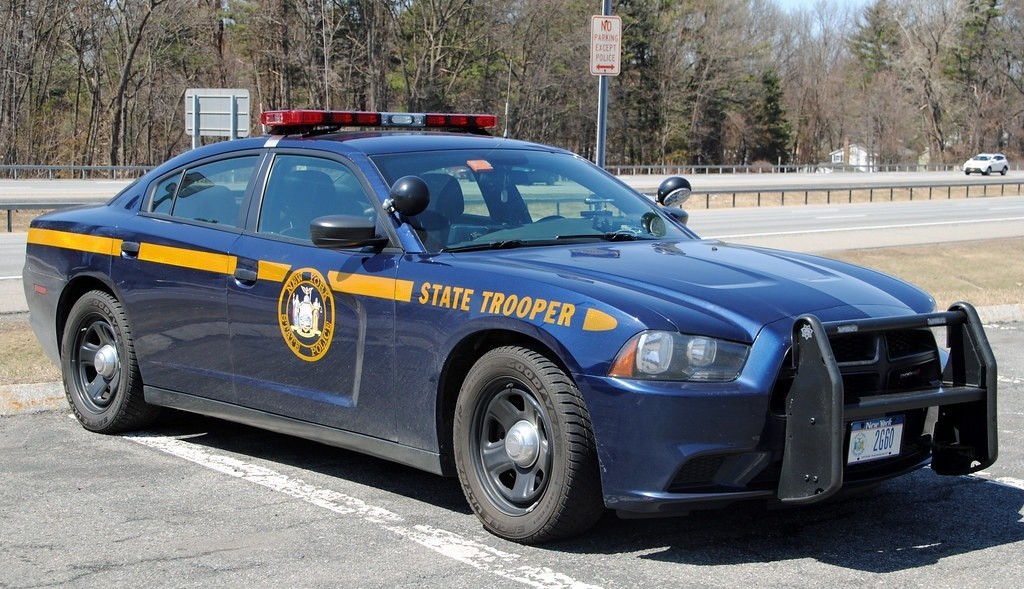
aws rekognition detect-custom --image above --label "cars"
[24,110,999,545]
[963,154,1010,176]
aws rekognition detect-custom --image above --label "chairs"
[269,170,337,234]
[414,173,466,225]
